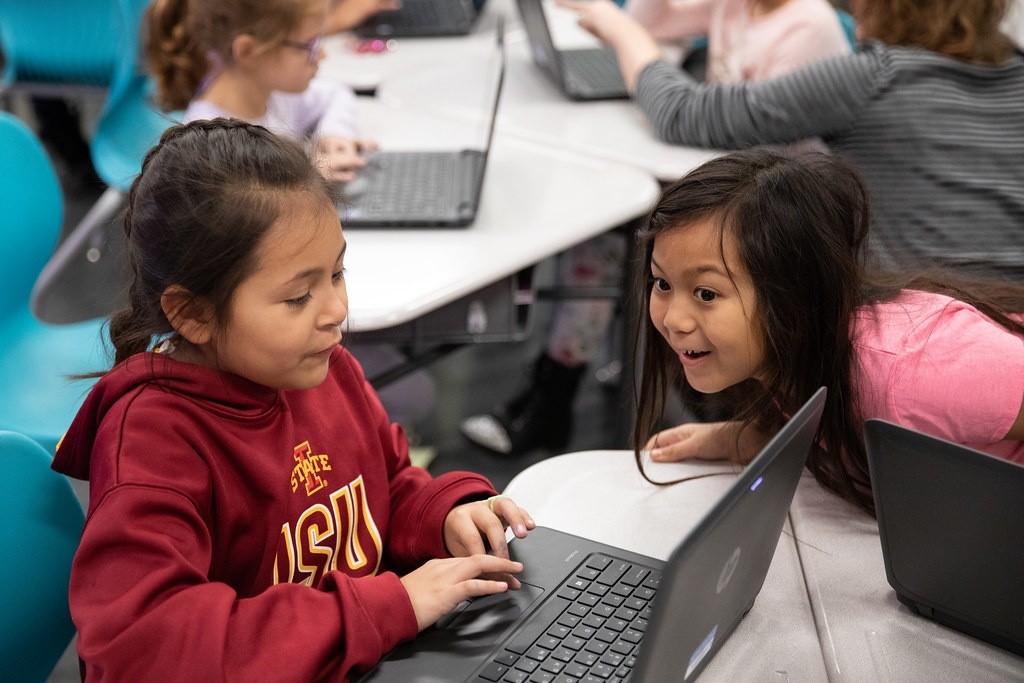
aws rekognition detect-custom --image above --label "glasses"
[283,37,321,65]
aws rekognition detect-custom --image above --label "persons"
[49,117,535,683]
[141,0,436,434]
[554,0,1024,310]
[459,0,853,461]
[633,138,1024,499]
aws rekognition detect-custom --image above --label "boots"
[460,348,590,456]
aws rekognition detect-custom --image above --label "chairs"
[1,1,183,453]
[1,431,88,683]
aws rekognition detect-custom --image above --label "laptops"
[863,419,1024,660]
[328,0,634,229]
[357,385,829,683]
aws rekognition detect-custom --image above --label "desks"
[499,450,1024,683]
[339,9,837,452]
